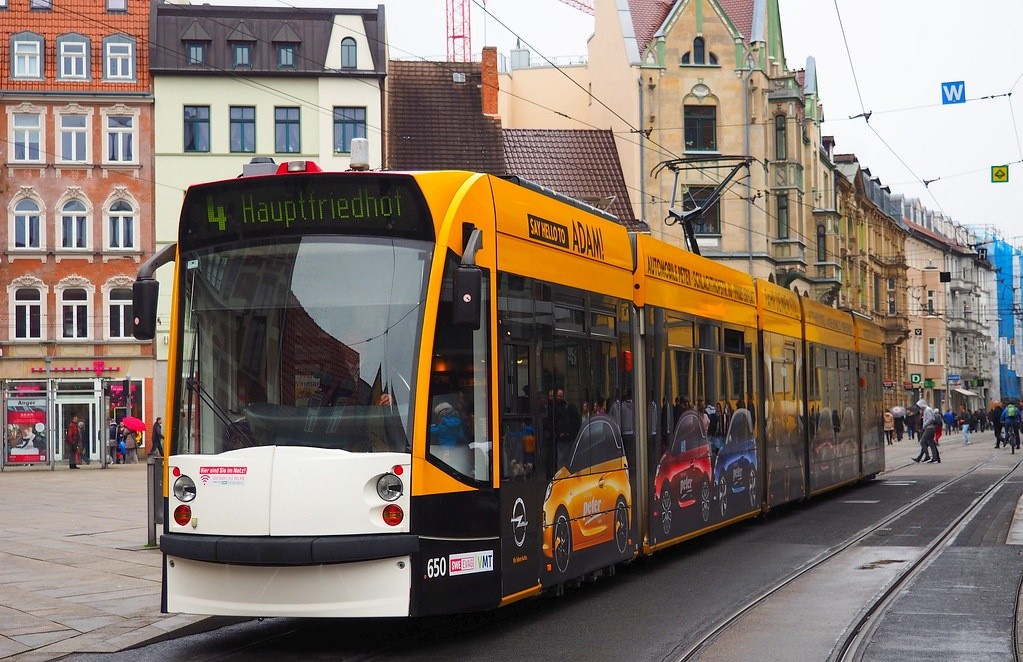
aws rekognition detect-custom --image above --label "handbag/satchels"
[110,440,117,447]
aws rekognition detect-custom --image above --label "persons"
[13,424,35,449]
[110,419,126,464]
[810,405,841,436]
[150,418,164,457]
[960,406,971,445]
[123,428,138,464]
[321,326,397,404]
[78,419,90,465]
[67,416,82,469]
[884,397,1023,463]
[508,387,634,480]
[916,399,940,463]
[651,392,755,461]
[136,431,141,444]
[430,402,464,447]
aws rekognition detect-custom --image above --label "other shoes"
[890,441,892,444]
[70,465,80,469]
[927,457,941,463]
[922,456,930,462]
[936,442,939,446]
[1016,445,1020,449]
[994,446,999,448]
[898,439,901,441]
[912,458,920,463]
[1003,441,1007,447]
[123,462,125,464]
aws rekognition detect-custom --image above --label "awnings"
[954,389,977,396]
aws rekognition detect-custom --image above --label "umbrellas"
[890,406,906,418]
[123,417,147,432]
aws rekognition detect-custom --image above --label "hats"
[434,402,452,418]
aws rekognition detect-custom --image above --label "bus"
[130,138,885,618]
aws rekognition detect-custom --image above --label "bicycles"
[1001,419,1021,454]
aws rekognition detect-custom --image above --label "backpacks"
[1006,404,1017,417]
